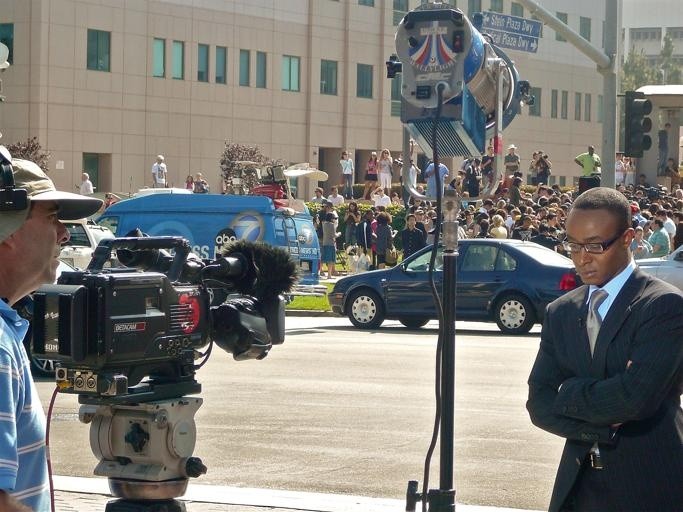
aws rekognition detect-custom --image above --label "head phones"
[1,162,28,211]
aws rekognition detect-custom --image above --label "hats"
[1,156,104,243]
[484,198,495,205]
[507,144,518,150]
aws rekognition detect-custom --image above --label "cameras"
[541,155,548,159]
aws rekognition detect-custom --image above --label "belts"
[583,450,609,472]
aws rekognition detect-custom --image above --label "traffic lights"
[624,90,653,157]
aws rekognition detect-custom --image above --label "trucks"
[95,193,327,306]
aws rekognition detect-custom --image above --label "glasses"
[561,235,622,254]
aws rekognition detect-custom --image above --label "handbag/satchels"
[384,248,399,265]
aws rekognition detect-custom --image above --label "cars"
[57,217,120,270]
[326,237,580,335]
[633,244,683,296]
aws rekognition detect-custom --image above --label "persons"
[340,152,354,200]
[80,172,93,196]
[0,157,105,512]
[309,173,683,279]
[616,155,631,185]
[658,123,671,175]
[665,158,680,177]
[152,155,167,188]
[525,187,683,512]
[184,173,209,194]
[575,145,601,177]
[364,146,495,206]
[504,144,551,186]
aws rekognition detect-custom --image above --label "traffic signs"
[478,28,538,53]
[480,11,543,38]
